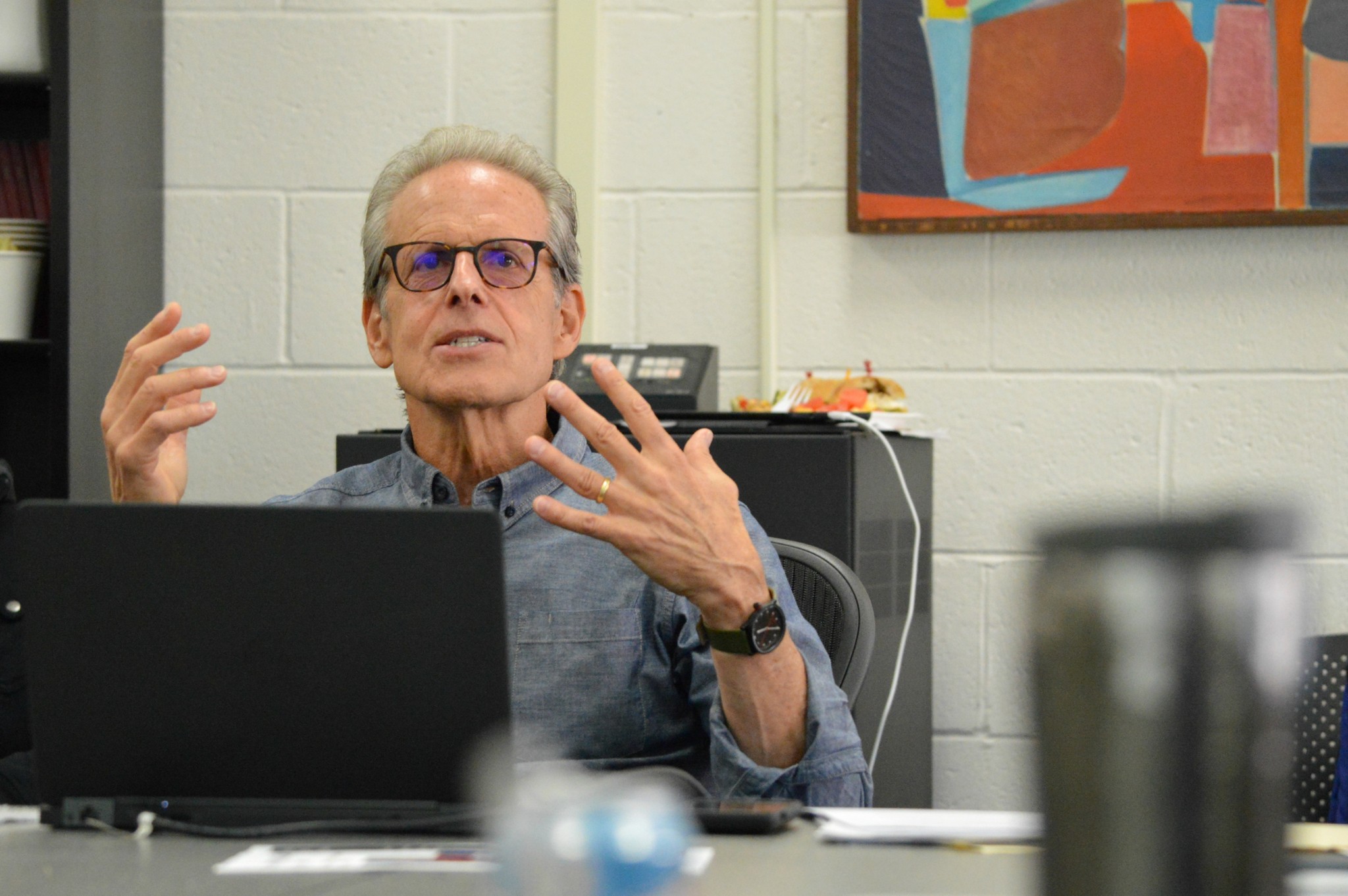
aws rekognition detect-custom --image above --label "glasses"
[371,237,568,292]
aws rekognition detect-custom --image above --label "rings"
[594,477,611,504]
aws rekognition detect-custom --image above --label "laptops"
[13,498,518,832]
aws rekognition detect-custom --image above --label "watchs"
[693,587,789,656]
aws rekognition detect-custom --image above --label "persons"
[96,122,881,808]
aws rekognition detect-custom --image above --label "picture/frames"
[846,0,1347,233]
